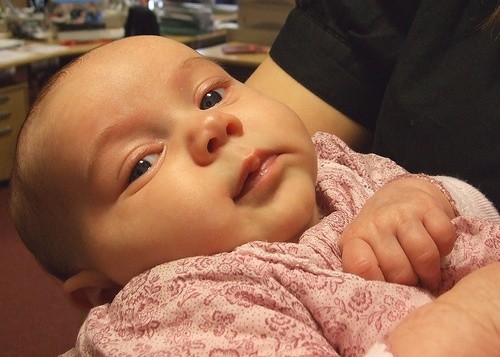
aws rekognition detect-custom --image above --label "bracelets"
[415,175,459,216]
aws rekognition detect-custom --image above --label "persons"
[243,0,499,217]
[10,33,500,356]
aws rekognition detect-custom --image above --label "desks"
[0,0,272,183]
